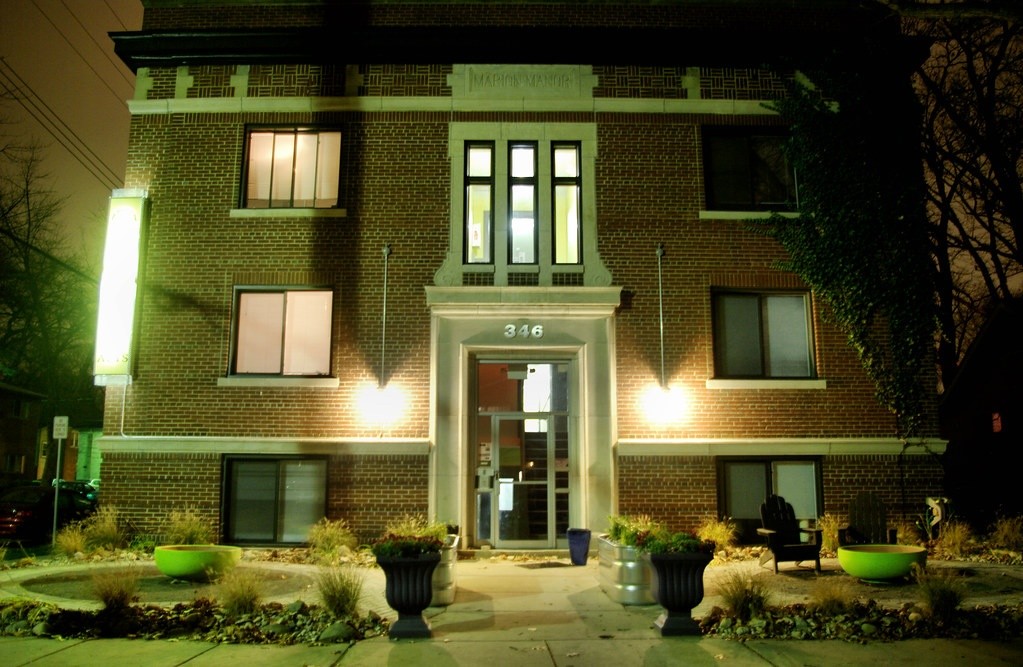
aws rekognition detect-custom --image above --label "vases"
[566,528,591,566]
[376,551,442,639]
[649,550,714,638]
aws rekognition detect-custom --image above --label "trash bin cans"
[567,527,592,566]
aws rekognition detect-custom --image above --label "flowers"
[372,512,453,559]
[635,530,717,563]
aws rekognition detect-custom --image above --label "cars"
[0,486,75,540]
[53,480,98,512]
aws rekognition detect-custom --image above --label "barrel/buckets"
[567,528,590,564]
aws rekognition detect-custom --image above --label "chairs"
[837,491,898,546]
[756,494,824,574]
